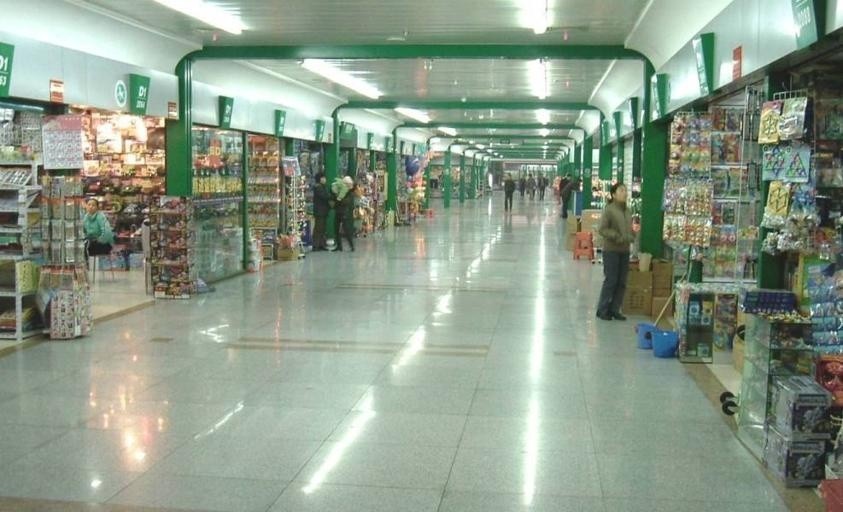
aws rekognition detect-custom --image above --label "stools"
[573,231,596,265]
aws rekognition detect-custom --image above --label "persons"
[505,173,515,212]
[518,174,548,200]
[559,174,571,217]
[592,187,598,191]
[561,179,582,218]
[83,198,114,270]
[311,172,361,252]
[596,183,636,320]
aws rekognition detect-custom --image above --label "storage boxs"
[620,259,675,319]
[563,209,579,252]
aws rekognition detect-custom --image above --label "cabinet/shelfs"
[660,74,842,510]
[1,97,247,341]
[589,128,642,262]
[247,130,427,271]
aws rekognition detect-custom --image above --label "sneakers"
[610,311,627,320]
[597,310,612,320]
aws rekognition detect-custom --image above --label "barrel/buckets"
[651,331,679,358]
[635,323,656,348]
